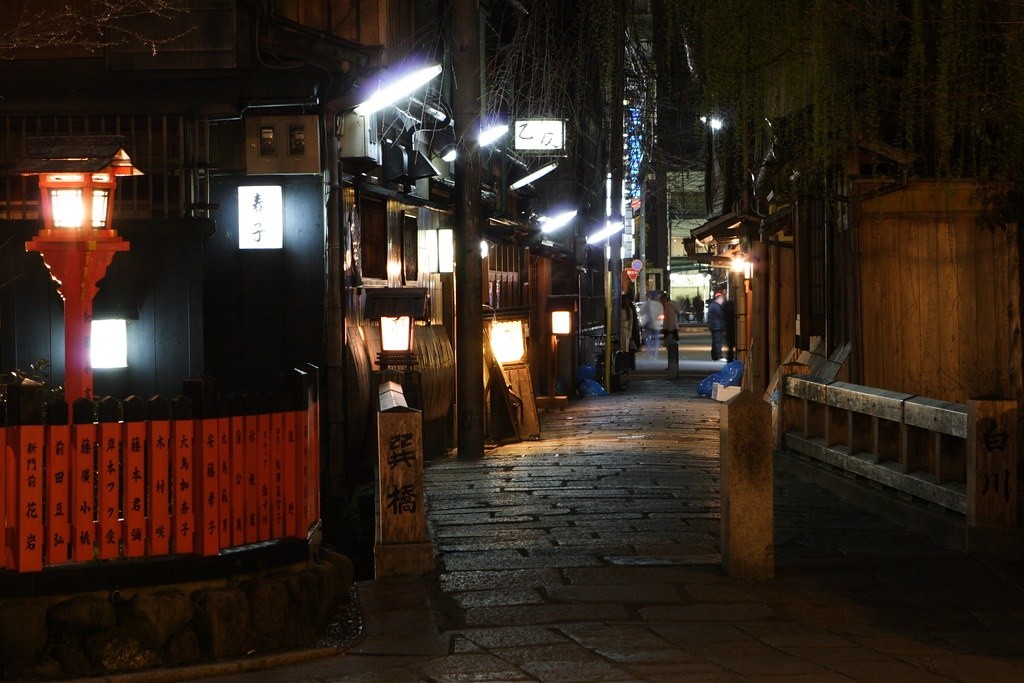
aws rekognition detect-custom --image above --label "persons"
[618,291,726,383]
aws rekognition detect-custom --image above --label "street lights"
[6,130,150,426]
